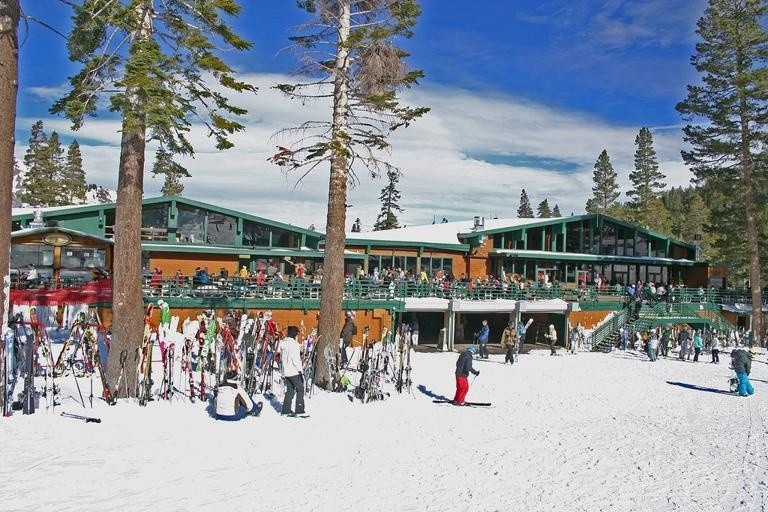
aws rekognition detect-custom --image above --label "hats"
[467,346,476,353]
[288,325,297,337]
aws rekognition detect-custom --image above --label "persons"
[543,324,557,355]
[240,266,249,280]
[477,320,491,359]
[214,370,263,420]
[732,345,754,396]
[248,271,267,286]
[193,266,211,290]
[501,320,518,364]
[416,270,510,299]
[277,325,310,417]
[55,302,65,332]
[339,311,354,362]
[221,268,228,286]
[157,300,171,329]
[151,267,163,297]
[345,264,417,297]
[570,329,579,353]
[593,273,768,364]
[294,264,325,284]
[511,271,553,296]
[579,281,586,290]
[451,345,479,407]
[177,269,184,288]
[26,263,38,283]
[273,273,282,282]
[517,321,525,355]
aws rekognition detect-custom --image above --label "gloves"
[473,370,480,375]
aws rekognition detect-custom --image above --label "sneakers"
[452,401,470,406]
[252,401,263,416]
[280,412,295,418]
[295,413,311,419]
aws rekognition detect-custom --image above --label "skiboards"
[0,304,414,418]
[433,401,491,408]
[518,318,533,339]
[0,304,414,415]
